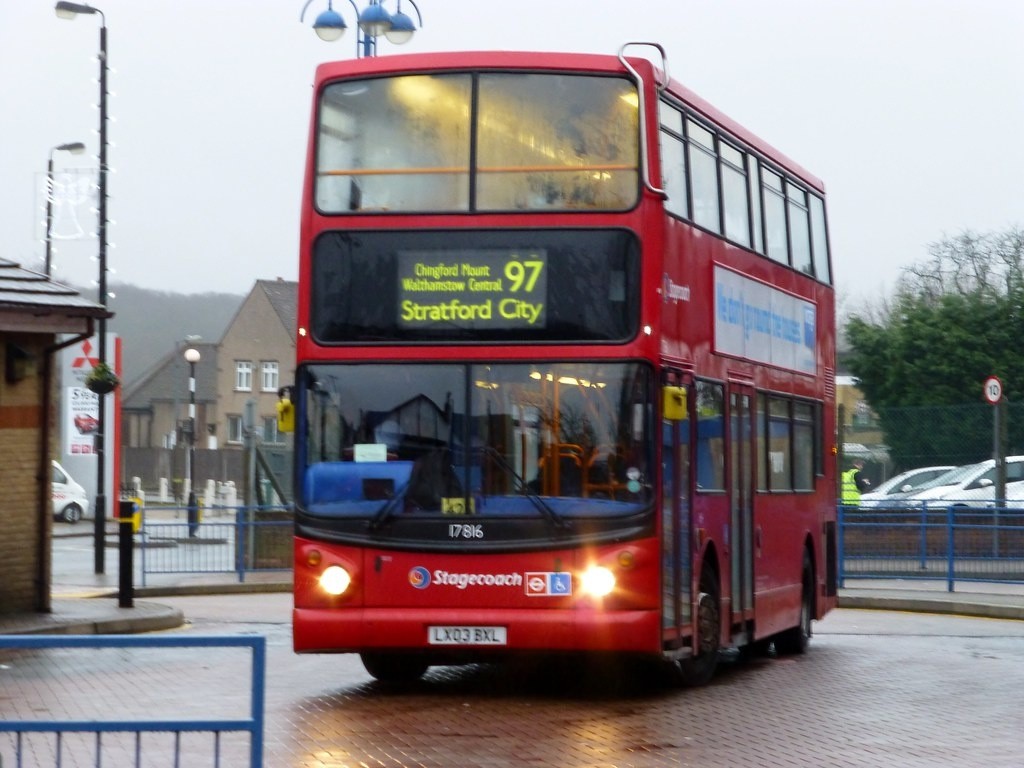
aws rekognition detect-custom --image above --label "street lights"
[43,141,85,278]
[292,0,427,57]
[55,1,107,575]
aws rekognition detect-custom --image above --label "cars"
[860,455,1024,510]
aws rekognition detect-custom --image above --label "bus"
[276,51,840,690]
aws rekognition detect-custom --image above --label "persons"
[387,413,460,514]
[841,459,870,505]
